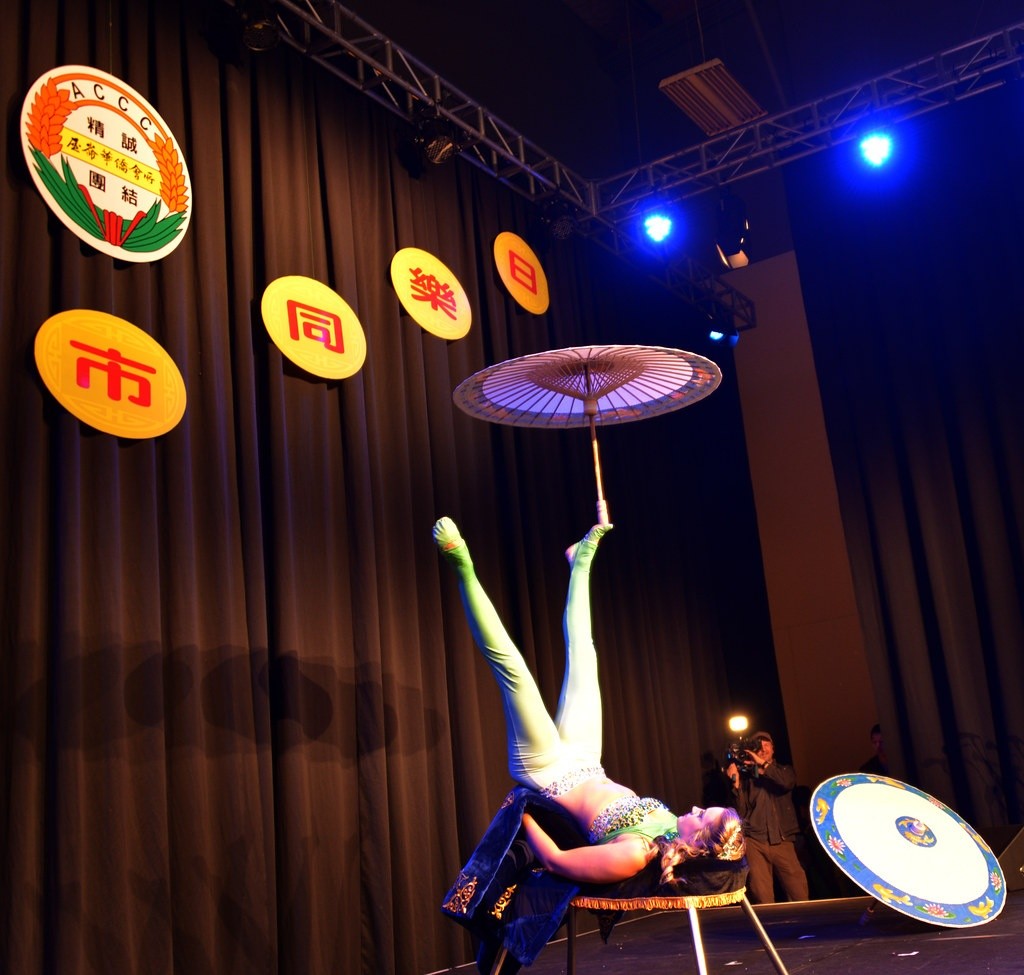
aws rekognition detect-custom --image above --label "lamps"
[659,57,768,138]
[713,205,751,269]
[397,117,467,183]
[540,194,578,241]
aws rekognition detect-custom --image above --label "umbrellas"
[453,344,724,524]
[809,772,1008,927]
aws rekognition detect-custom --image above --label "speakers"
[997,826,1024,891]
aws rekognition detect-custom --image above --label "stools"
[502,789,790,975]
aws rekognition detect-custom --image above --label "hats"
[749,731,773,744]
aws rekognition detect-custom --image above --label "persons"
[857,722,891,779]
[431,517,748,975]
[727,731,808,903]
